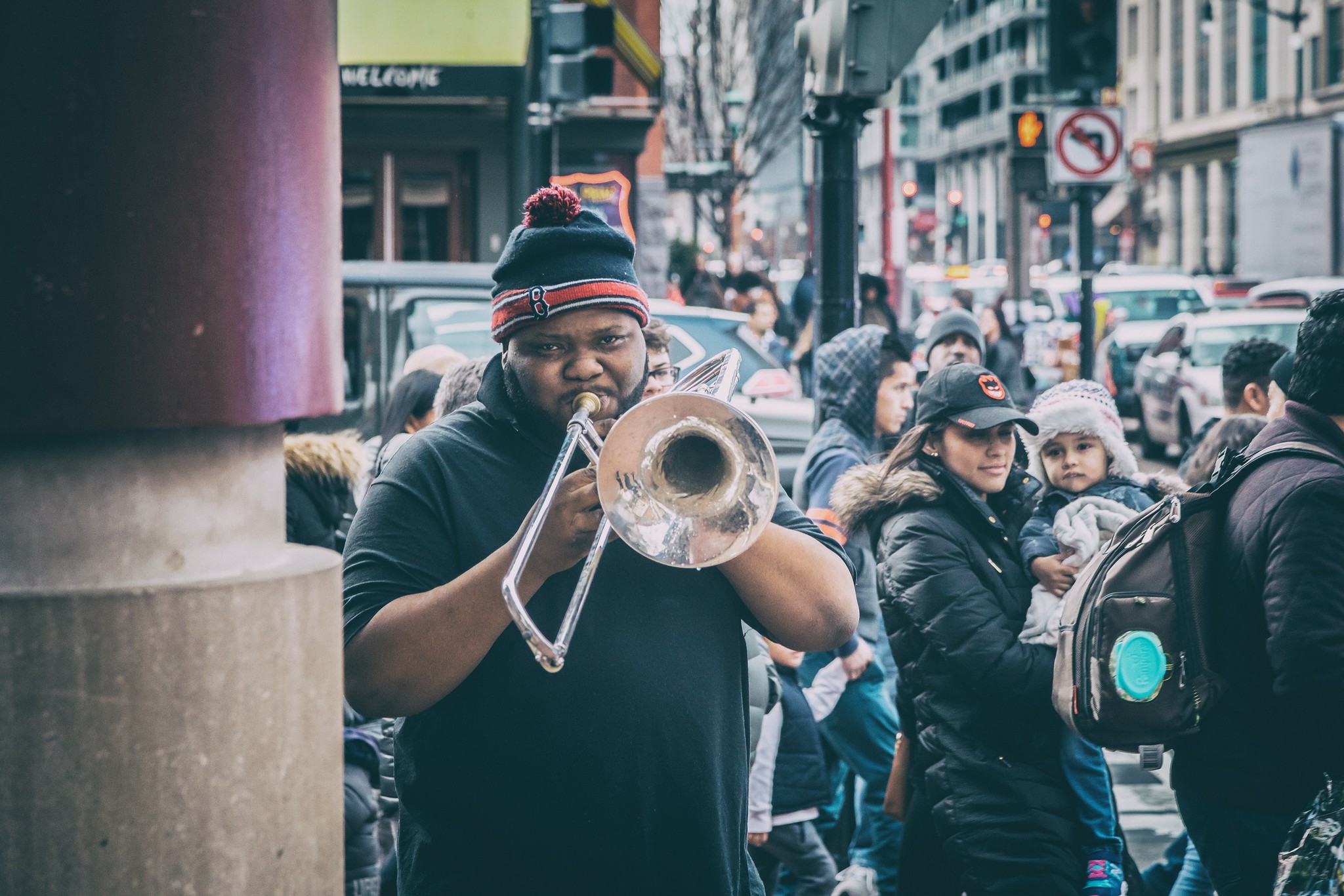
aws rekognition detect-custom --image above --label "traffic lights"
[1007,111,1047,156]
[548,2,621,105]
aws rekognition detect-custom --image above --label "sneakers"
[1081,859,1127,896]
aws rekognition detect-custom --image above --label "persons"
[1166,290,1344,896]
[288,208,1344,896]
[346,189,858,892]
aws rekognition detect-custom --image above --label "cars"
[1136,307,1318,471]
[332,233,1344,510]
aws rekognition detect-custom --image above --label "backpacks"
[1051,442,1344,771]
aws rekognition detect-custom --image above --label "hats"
[1014,379,1184,495]
[924,307,984,363]
[492,185,649,342]
[915,362,1040,437]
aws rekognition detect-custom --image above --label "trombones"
[498,346,780,674]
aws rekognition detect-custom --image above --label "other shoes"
[831,864,879,896]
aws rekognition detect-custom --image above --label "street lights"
[1200,2,1314,103]
